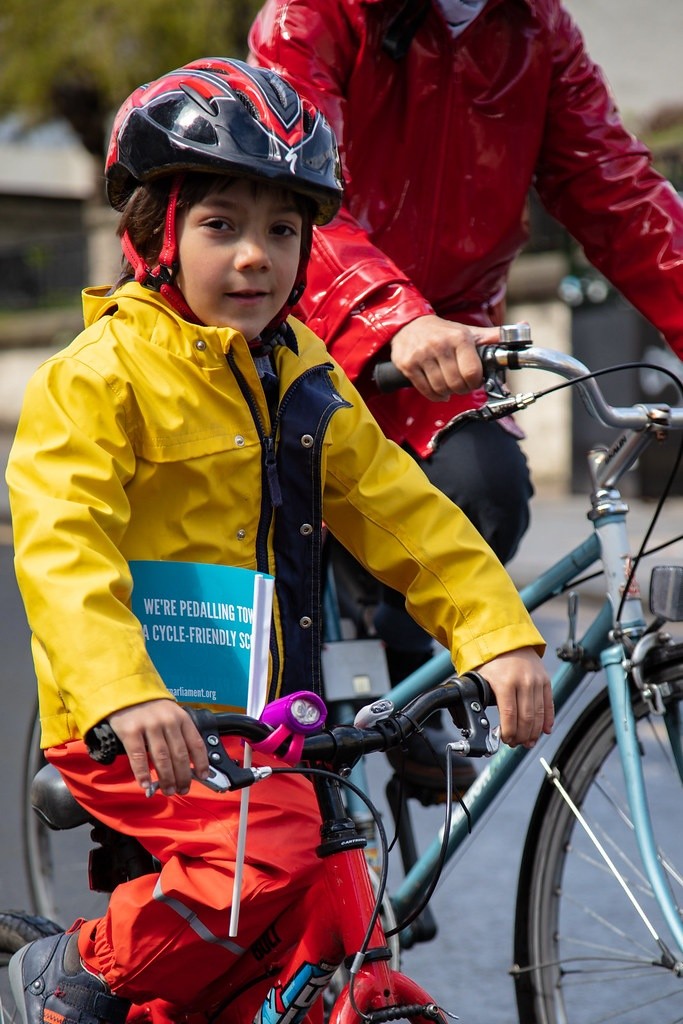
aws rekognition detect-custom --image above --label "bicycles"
[20,326,683,1017]
[3,669,508,1022]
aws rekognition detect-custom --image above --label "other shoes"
[385,726,478,802]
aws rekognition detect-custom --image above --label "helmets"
[104,58,345,226]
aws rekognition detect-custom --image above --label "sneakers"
[9,918,132,1024]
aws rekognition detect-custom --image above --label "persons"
[6,57,553,1024]
[251,0,683,807]
